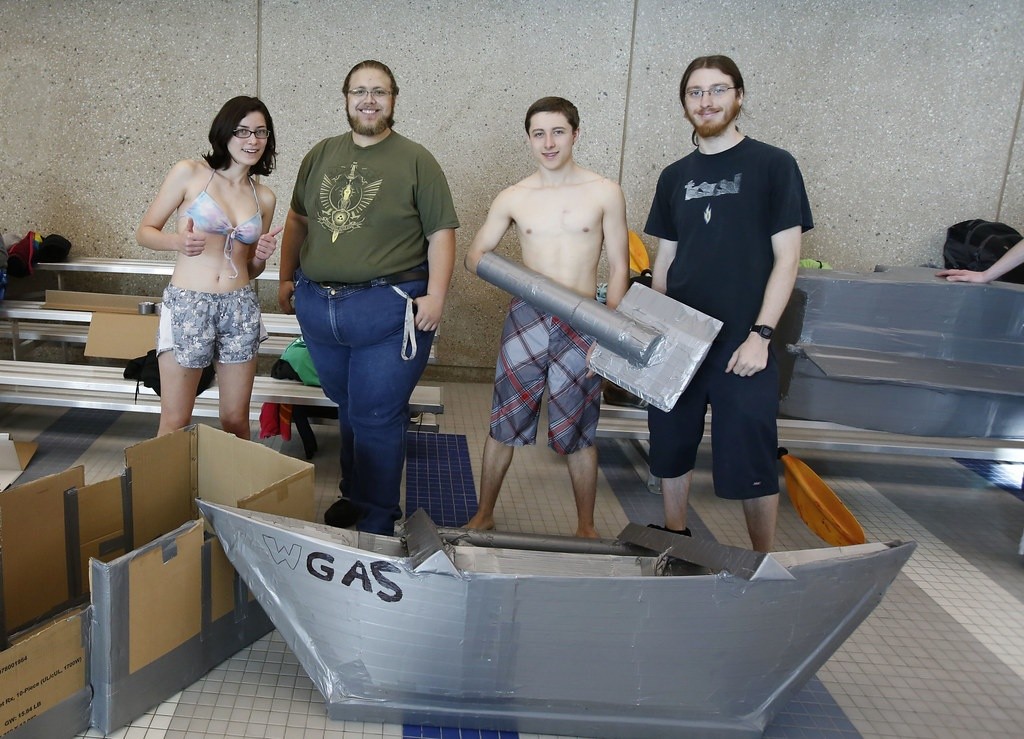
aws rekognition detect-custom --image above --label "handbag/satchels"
[943,219,1024,284]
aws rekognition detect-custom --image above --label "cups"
[139,300,163,315]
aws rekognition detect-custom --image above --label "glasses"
[685,86,735,99]
[232,129,270,139]
[347,89,392,98]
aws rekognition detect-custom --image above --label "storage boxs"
[0,424,314,739]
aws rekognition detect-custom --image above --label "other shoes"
[324,499,362,528]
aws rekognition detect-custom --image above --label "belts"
[319,268,428,288]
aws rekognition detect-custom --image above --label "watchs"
[749,323,776,341]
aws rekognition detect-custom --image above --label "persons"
[464,95,630,538]
[642,55,813,557]
[277,59,460,537]
[134,96,283,441]
[936,238,1024,283]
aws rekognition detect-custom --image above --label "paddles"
[627,229,866,547]
[475,248,725,413]
